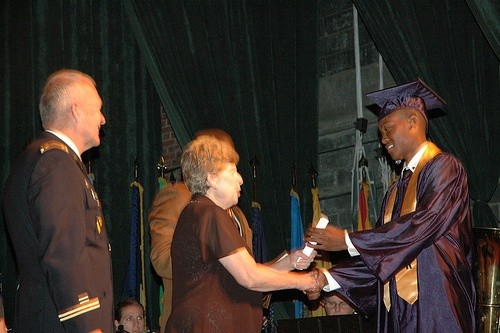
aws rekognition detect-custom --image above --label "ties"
[392,167,412,222]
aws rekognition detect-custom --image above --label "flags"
[250,199,278,333]
[121,165,149,333]
[308,172,334,316]
[356,144,375,232]
[290,189,308,318]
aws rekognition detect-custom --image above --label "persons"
[162,135,317,333]
[113,298,151,333]
[13,69,111,332]
[301,97,478,333]
[149,128,254,333]
[320,292,355,316]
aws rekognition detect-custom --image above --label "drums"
[472,227,500,333]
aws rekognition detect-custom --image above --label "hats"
[365,77,447,134]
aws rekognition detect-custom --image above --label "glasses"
[324,300,352,309]
